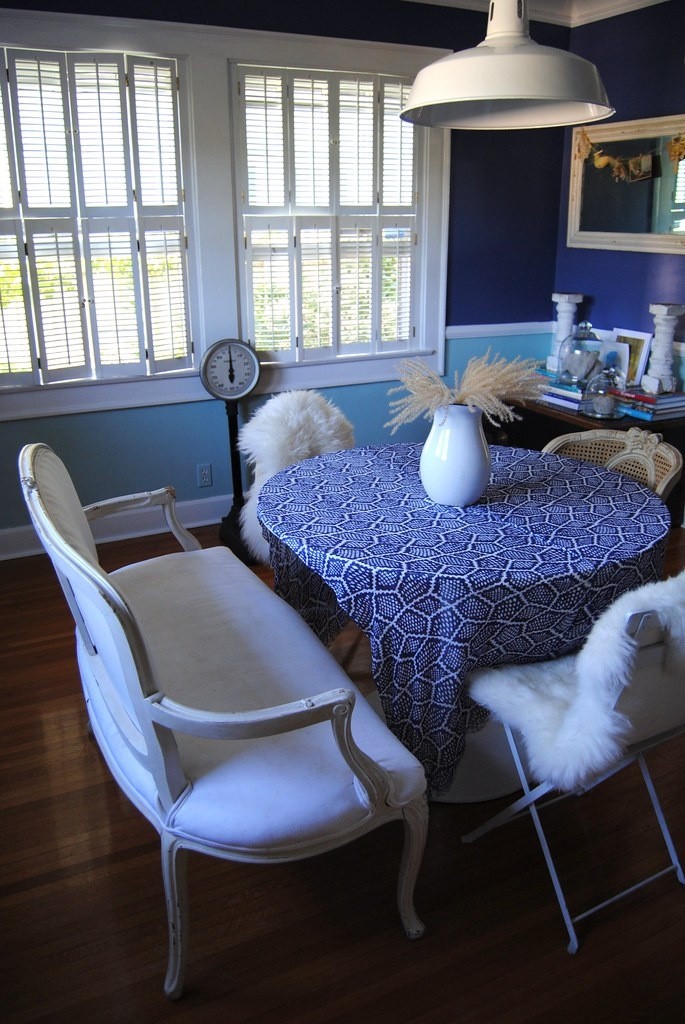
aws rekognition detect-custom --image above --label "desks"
[258,443,670,804]
[498,398,685,446]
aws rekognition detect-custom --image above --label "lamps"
[400,0,616,130]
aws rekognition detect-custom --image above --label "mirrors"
[566,114,685,256]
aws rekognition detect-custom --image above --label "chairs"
[361,569,685,955]
[542,427,683,501]
[235,389,355,566]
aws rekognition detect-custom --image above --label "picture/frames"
[611,328,653,386]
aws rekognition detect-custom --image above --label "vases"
[420,404,491,507]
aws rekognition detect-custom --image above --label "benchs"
[18,443,427,1000]
